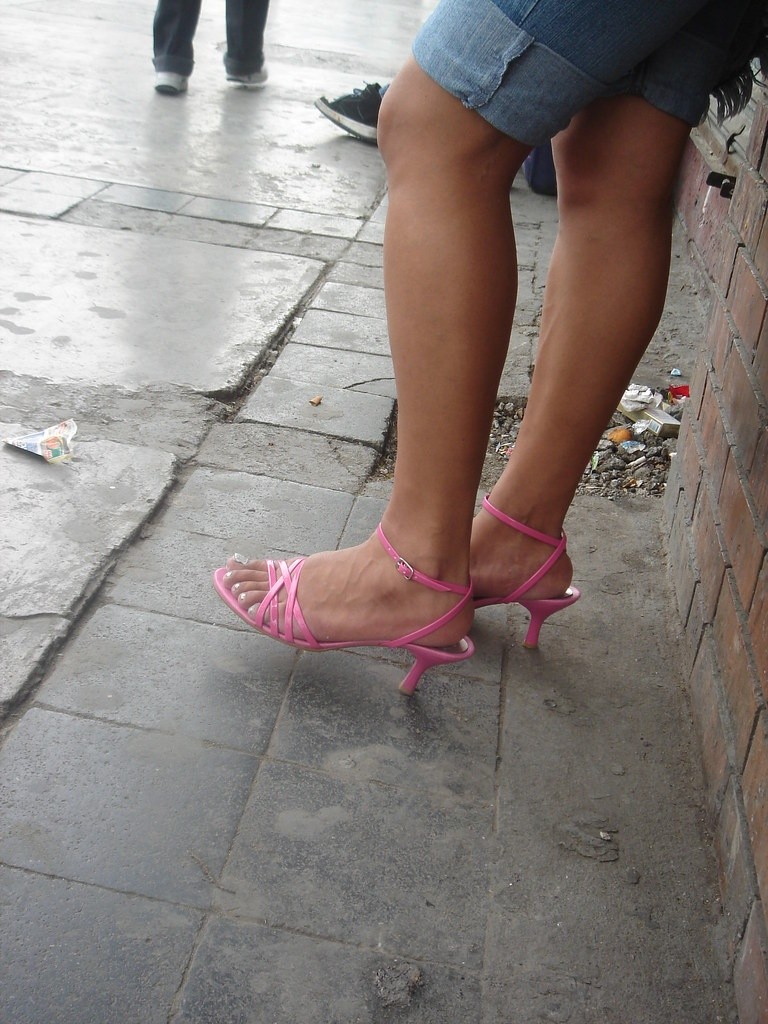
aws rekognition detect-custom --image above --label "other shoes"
[154,71,187,93]
[225,68,269,89]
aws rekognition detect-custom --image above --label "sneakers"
[315,81,382,144]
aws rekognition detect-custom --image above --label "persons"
[152,0,271,94]
[212,0,768,695]
[315,80,557,197]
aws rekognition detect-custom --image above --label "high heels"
[467,494,581,649]
[212,523,474,696]
